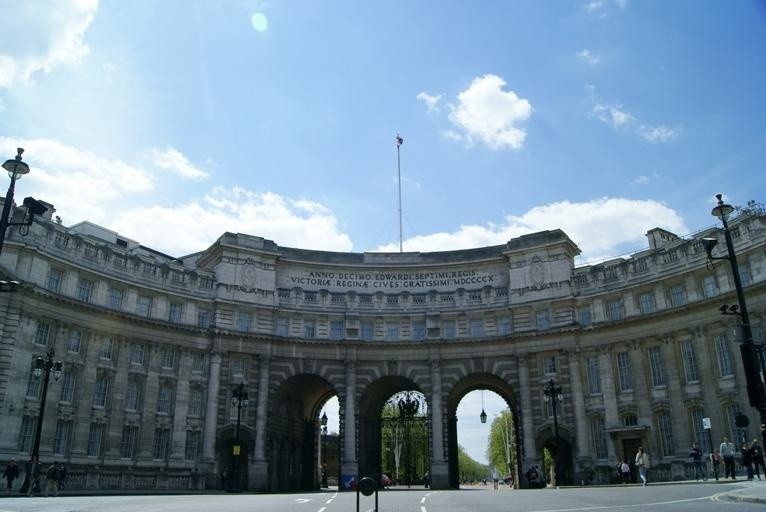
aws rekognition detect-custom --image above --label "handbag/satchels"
[634,456,645,466]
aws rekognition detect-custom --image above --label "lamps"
[318,405,328,425]
[480,390,487,423]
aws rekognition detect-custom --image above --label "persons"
[508,472,514,487]
[423,469,430,488]
[635,446,652,487]
[711,437,766,481]
[492,469,500,491]
[688,440,707,482]
[525,464,546,488]
[759,423,766,458]
[616,458,638,485]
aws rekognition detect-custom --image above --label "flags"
[396,135,404,149]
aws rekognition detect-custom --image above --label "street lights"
[701,192,765,448]
[323,427,329,488]
[0,147,49,248]
[20,345,64,494]
[225,381,249,493]
[543,377,563,486]
